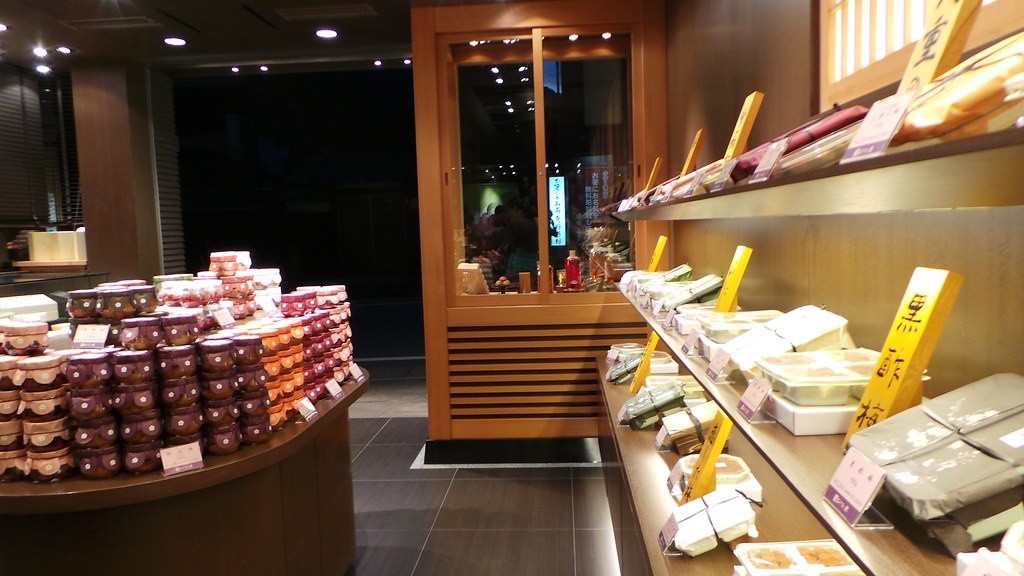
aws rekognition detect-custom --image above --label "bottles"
[565,249,582,290]
[0,252,354,478]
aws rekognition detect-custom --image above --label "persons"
[487,197,538,277]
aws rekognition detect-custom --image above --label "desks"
[0,362,369,576]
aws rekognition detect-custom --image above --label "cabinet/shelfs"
[596,122,1024,576]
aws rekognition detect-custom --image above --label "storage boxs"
[28,230,87,261]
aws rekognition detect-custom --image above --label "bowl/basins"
[694,309,931,404]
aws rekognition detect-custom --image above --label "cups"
[556,269,567,286]
